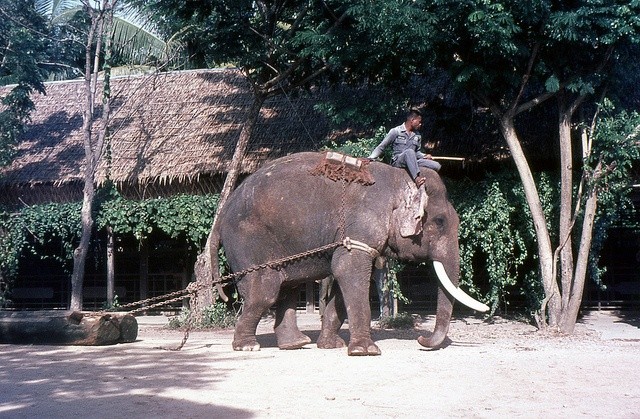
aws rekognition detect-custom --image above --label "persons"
[368,110,453,188]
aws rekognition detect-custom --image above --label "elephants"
[208,148,492,357]
